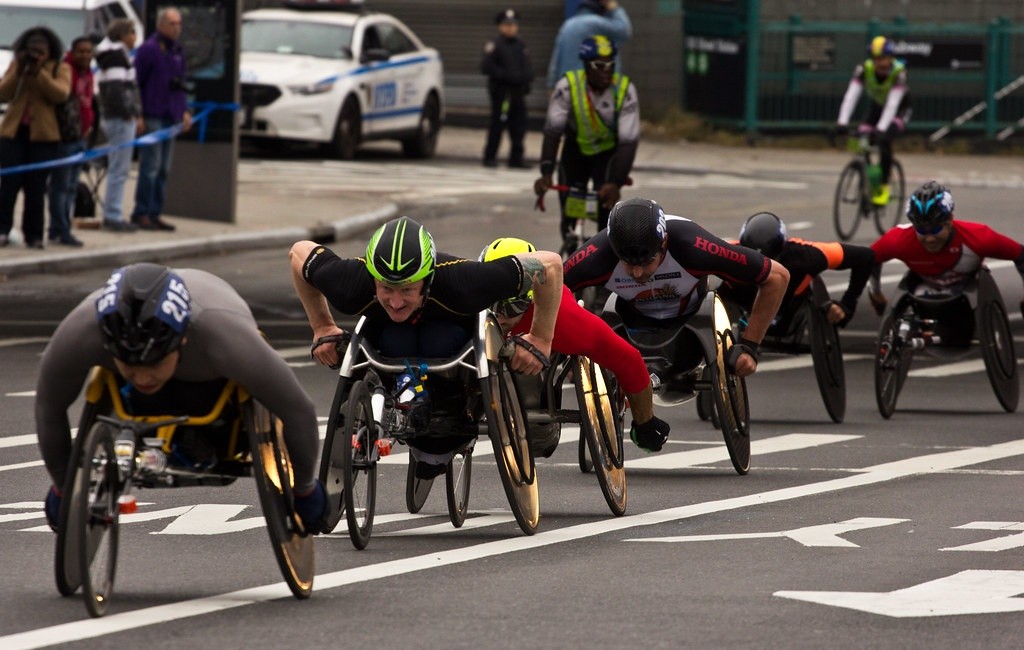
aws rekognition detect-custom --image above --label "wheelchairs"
[578,286,752,476]
[405,347,628,529]
[695,275,846,430]
[874,262,1019,420]
[53,363,316,619]
[316,306,541,551]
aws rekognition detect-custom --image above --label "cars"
[235,6,446,163]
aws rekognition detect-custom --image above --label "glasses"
[621,253,660,266]
[914,224,943,236]
[129,29,135,35]
[488,299,529,319]
[591,60,615,72]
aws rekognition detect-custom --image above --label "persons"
[34,261,330,536]
[289,214,563,377]
[866,180,1024,317]
[477,238,670,453]
[131,5,195,231]
[534,0,642,256]
[563,196,791,377]
[829,36,913,206]
[481,8,532,170]
[727,211,875,328]
[0,28,73,250]
[94,17,141,229]
[48,31,111,245]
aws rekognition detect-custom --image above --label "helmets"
[478,237,537,304]
[607,197,667,262]
[365,216,438,286]
[739,212,790,260]
[906,181,955,226]
[578,35,619,61]
[94,262,190,367]
[867,36,895,60]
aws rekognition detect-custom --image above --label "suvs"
[0,0,146,124]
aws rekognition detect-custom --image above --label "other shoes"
[0,235,9,248]
[506,160,534,169]
[25,237,44,250]
[47,230,83,248]
[871,185,895,207]
[103,218,137,232]
[131,210,176,231]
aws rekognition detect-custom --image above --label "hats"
[496,9,519,24]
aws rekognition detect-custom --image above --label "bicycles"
[827,124,907,242]
[532,176,633,265]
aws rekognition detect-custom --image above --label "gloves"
[45,484,92,536]
[628,415,671,452]
[293,479,330,538]
